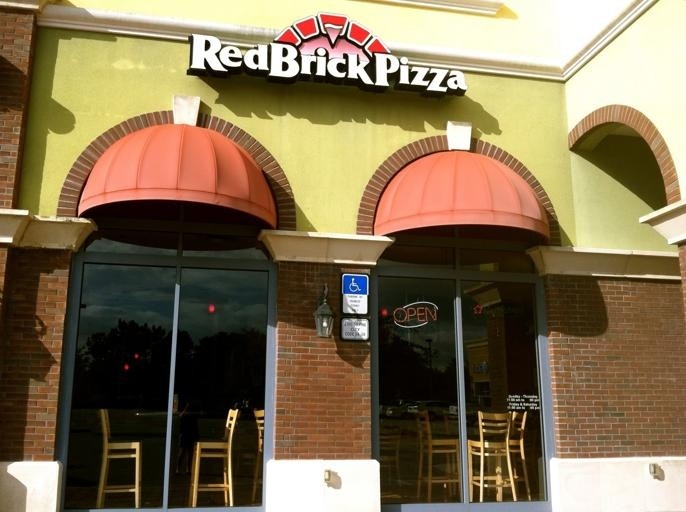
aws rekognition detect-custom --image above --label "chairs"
[380,407,534,501]
[188,406,263,509]
[95,406,143,509]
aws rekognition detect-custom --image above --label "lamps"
[311,283,338,340]
